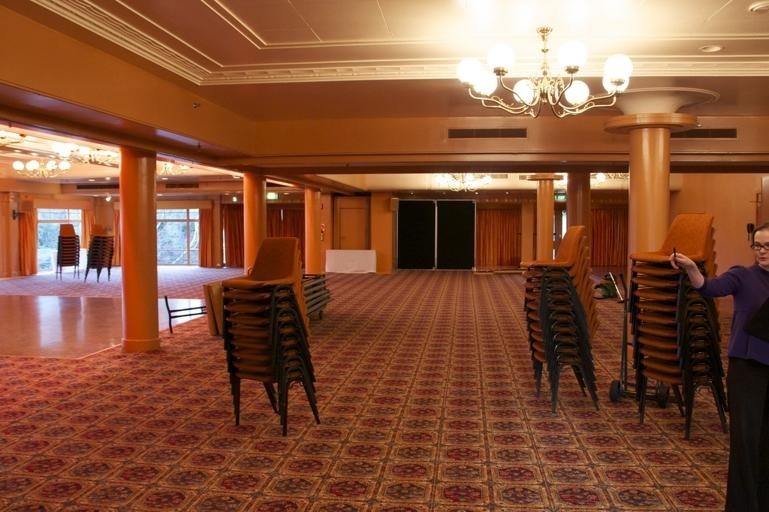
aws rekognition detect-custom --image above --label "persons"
[668,222,769,512]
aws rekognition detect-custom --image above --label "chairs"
[56,224,114,283]
[520,224,603,414]
[629,217,728,441]
[222,236,321,436]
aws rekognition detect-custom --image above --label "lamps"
[444,23,632,118]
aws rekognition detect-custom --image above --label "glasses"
[750,244,769,250]
